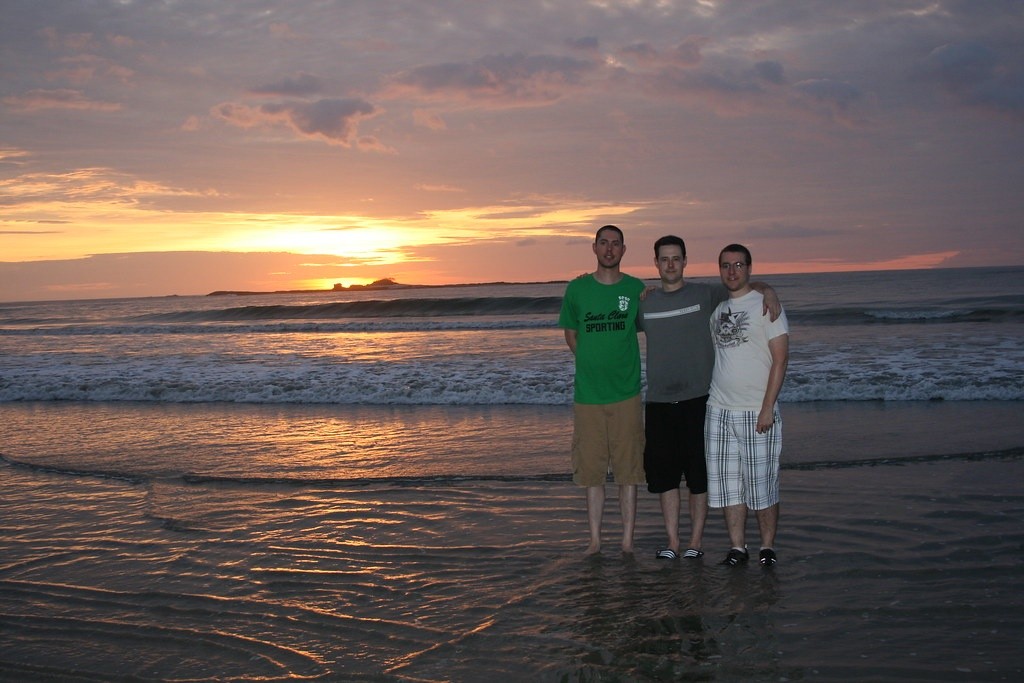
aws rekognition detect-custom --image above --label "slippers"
[656,549,680,560]
[683,548,704,559]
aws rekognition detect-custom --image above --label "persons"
[557,225,647,555]
[576,235,782,559]
[640,244,790,567]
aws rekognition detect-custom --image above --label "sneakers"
[721,548,749,568]
[759,549,776,565]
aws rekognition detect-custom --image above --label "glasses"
[720,262,748,270]
